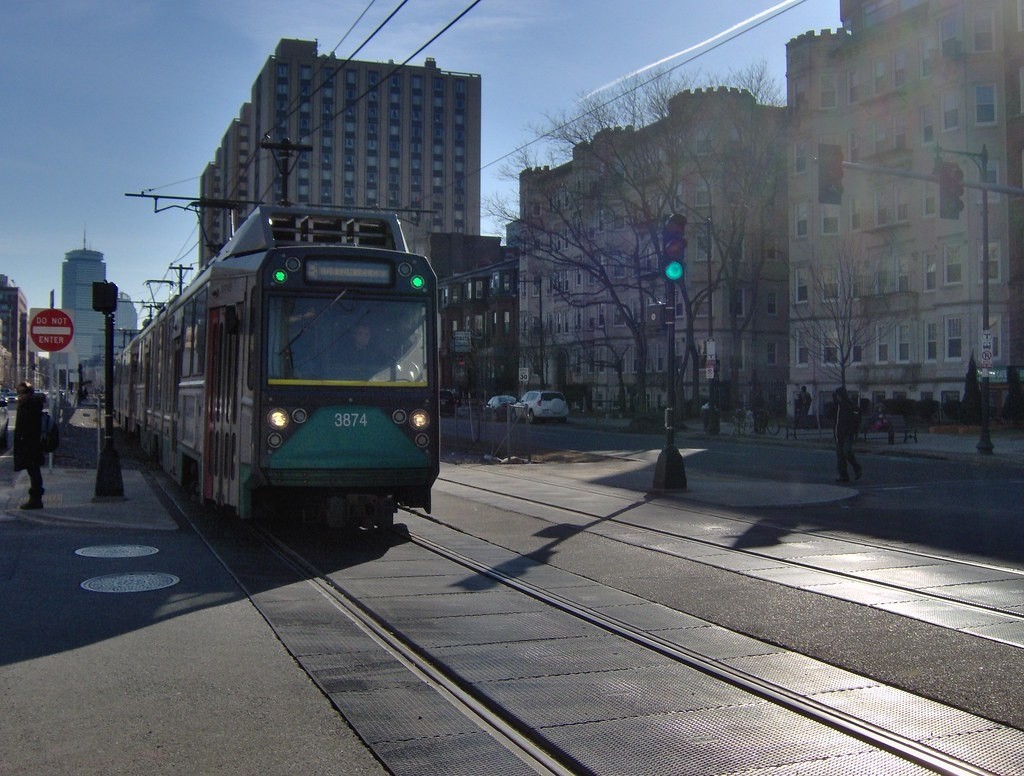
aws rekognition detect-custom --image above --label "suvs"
[439,389,456,418]
[510,390,570,424]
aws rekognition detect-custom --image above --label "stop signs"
[27,308,75,352]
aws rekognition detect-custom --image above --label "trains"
[109,199,443,549]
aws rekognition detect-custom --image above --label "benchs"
[861,415,918,444]
[786,415,836,440]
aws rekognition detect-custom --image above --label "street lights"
[931,143,998,456]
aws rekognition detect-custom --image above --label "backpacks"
[38,412,60,452]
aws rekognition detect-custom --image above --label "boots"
[20,488,45,509]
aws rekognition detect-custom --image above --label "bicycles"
[727,408,780,435]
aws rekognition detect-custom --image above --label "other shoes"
[835,478,849,484]
[855,471,862,479]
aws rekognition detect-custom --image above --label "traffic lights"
[660,213,688,283]
[938,160,966,221]
[817,142,844,206]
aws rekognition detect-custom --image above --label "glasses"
[17,389,29,397]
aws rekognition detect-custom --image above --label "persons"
[871,406,894,445]
[833,385,863,482]
[13,381,47,509]
[336,320,384,381]
[800,385,811,415]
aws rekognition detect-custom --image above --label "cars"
[482,395,519,422]
[0,388,57,453]
